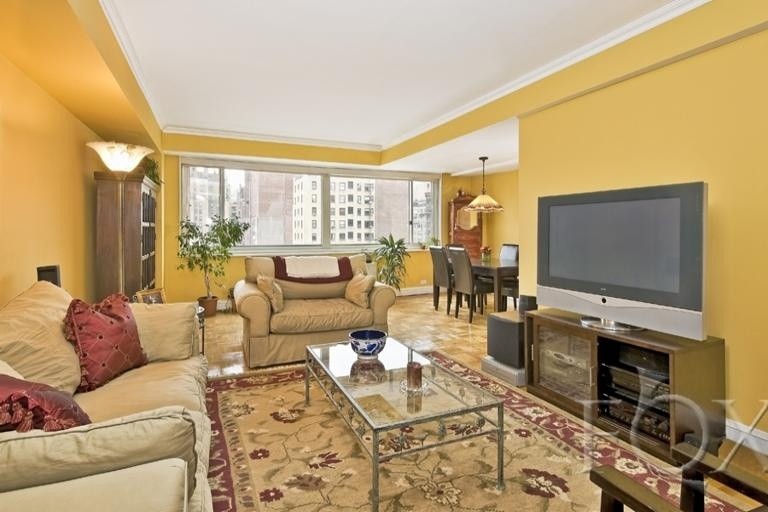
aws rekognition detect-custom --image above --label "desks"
[449,258,517,314]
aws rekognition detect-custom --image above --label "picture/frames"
[134,288,166,303]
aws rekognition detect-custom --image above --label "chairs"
[500,280,518,310]
[429,246,469,315]
[499,244,518,265]
[448,246,493,323]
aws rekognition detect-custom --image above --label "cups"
[407,395,423,414]
[406,362,422,387]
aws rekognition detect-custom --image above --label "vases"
[481,253,491,263]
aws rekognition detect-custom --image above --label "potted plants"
[175,212,251,317]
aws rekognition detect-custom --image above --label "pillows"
[256,274,284,313]
[62,291,148,392]
[344,270,375,308]
[0,374,92,432]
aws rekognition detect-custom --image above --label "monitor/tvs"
[536,182,708,343]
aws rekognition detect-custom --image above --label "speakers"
[519,295,536,313]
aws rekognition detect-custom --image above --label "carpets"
[205,350,754,512]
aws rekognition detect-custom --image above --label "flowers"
[480,246,491,254]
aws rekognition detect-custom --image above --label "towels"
[282,255,341,280]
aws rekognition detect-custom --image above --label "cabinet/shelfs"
[93,171,160,297]
[448,190,484,259]
[524,308,725,468]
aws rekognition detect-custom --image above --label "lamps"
[85,141,154,295]
[463,157,504,213]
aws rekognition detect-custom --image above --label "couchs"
[233,254,395,369]
[0,280,214,511]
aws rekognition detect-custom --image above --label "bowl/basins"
[349,328,387,359]
[349,360,388,385]
